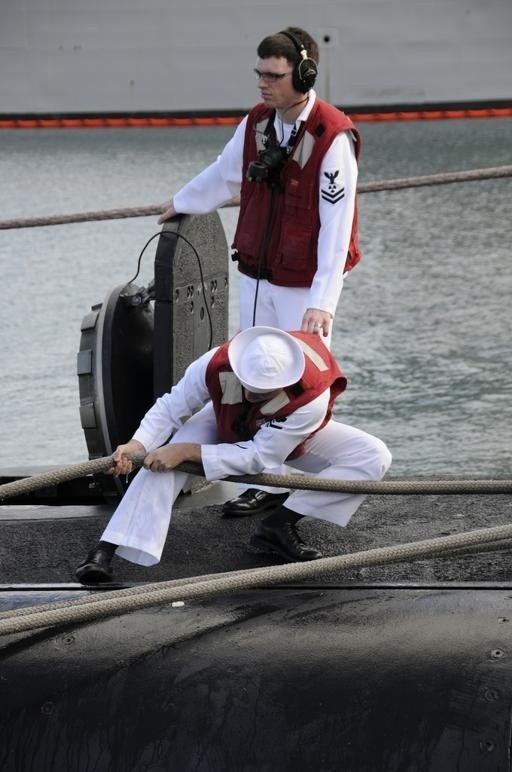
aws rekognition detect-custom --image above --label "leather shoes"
[248,519,325,563]
[220,484,290,517]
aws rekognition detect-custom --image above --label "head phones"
[279,30,318,92]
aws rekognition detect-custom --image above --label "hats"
[226,324,308,396]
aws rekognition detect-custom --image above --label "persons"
[154,23,366,519]
[73,327,396,585]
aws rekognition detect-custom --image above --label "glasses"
[250,65,294,83]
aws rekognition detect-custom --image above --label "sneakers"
[72,546,115,587]
